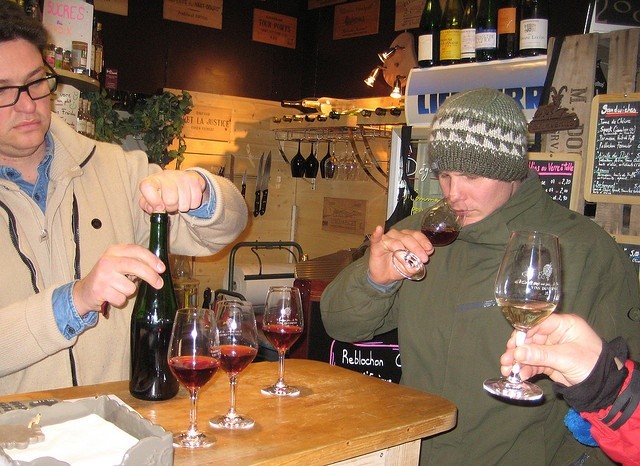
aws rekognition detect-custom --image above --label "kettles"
[414,0,444,68]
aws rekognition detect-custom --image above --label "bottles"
[361,109,377,124]
[458,0,476,65]
[76,97,84,134]
[304,113,322,127]
[374,108,393,123]
[127,214,182,400]
[292,113,310,127]
[495,0,519,61]
[91,21,106,81]
[82,99,89,134]
[470,0,497,62]
[103,64,119,99]
[316,114,335,127]
[301,96,356,117]
[280,96,318,115]
[282,113,297,128]
[56,48,63,70]
[518,0,551,58]
[91,114,97,138]
[86,101,92,138]
[390,108,407,123]
[329,108,361,127]
[438,0,460,68]
[592,58,609,98]
[45,44,55,66]
[62,50,71,68]
[272,115,289,127]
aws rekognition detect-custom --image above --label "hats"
[426,87,531,182]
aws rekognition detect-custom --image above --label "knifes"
[251,152,266,217]
[260,149,272,217]
[240,169,248,200]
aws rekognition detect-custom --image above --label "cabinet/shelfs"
[273,112,407,130]
[402,56,549,217]
[49,69,103,134]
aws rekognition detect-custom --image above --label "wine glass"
[324,136,338,181]
[347,138,359,180]
[318,138,335,179]
[337,137,351,180]
[165,305,223,453]
[290,140,305,178]
[392,197,468,282]
[259,285,304,400]
[208,299,259,430]
[482,230,561,400]
[358,138,374,184]
[305,141,318,178]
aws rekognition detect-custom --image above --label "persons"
[318,88,639,466]
[499,312,639,465]
[0,1,248,397]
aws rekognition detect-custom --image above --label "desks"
[0,358,458,466]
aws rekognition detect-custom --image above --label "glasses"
[0,57,58,108]
